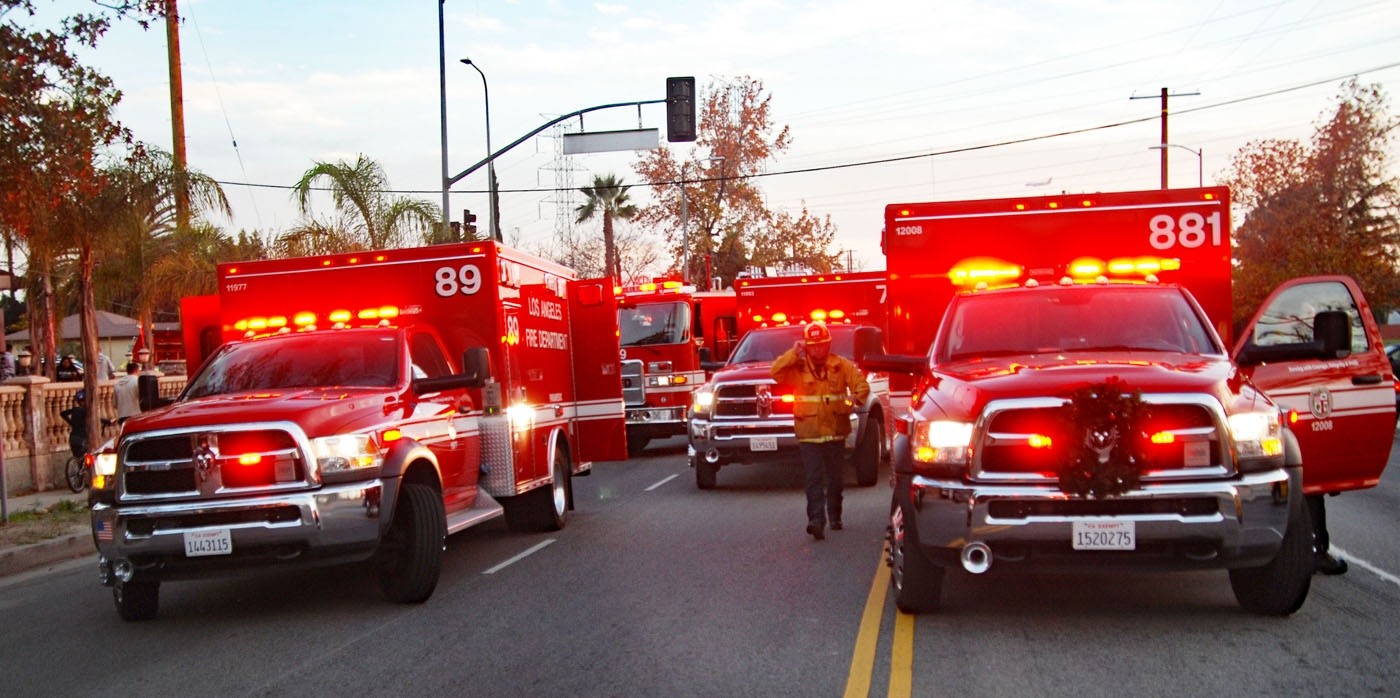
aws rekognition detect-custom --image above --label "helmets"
[804,322,832,344]
[76,391,86,401]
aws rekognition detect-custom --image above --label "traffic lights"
[463,209,477,241]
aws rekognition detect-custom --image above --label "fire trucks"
[849,184,1395,619]
[615,268,918,493]
[85,236,630,624]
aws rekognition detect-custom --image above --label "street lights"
[459,57,498,240]
[681,156,726,286]
[1147,144,1203,187]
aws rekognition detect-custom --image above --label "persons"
[768,321,870,541]
[5,344,114,382]
[111,363,140,438]
[1279,298,1349,575]
[60,389,112,470]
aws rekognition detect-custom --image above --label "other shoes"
[829,512,842,530]
[806,520,824,539]
[1314,551,1346,575]
